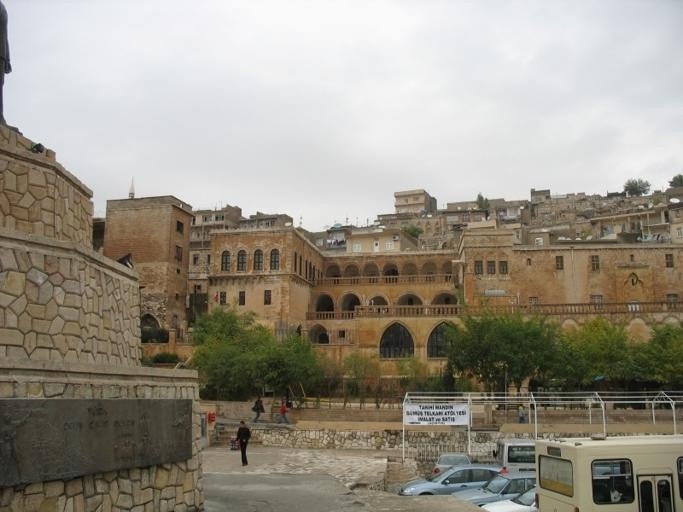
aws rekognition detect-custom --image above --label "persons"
[518,405,526,423]
[276,403,289,423]
[251,396,264,422]
[233,419,250,466]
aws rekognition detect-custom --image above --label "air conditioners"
[391,234,400,241]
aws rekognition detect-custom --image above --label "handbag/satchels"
[231,439,242,450]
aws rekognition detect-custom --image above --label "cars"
[397,438,536,512]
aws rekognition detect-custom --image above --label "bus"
[536,435,682,512]
